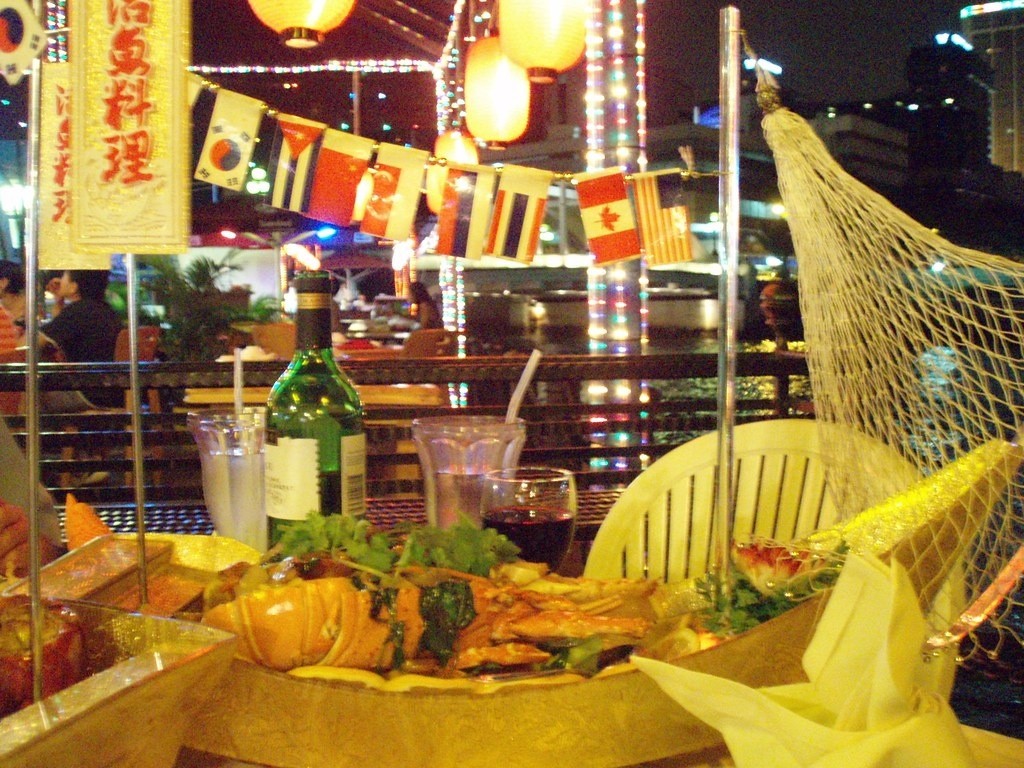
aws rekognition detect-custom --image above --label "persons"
[407,281,444,329]
[0,260,124,487]
[0,416,65,580]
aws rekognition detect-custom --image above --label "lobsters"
[199,551,661,679]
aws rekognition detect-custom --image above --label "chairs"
[581,417,963,704]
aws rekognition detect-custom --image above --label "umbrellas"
[294,250,392,281]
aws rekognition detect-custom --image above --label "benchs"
[2,360,778,543]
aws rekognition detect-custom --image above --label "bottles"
[260,270,367,550]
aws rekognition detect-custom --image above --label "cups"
[187,407,271,554]
[476,468,578,571]
[407,414,527,530]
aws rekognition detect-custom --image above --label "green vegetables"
[694,542,849,639]
[261,513,521,675]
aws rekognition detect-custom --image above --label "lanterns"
[250,0,587,217]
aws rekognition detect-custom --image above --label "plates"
[112,533,265,580]
[103,563,228,625]
[0,438,1023,768]
[0,593,241,768]
[0,534,175,605]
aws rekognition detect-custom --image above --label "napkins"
[627,549,975,768]
